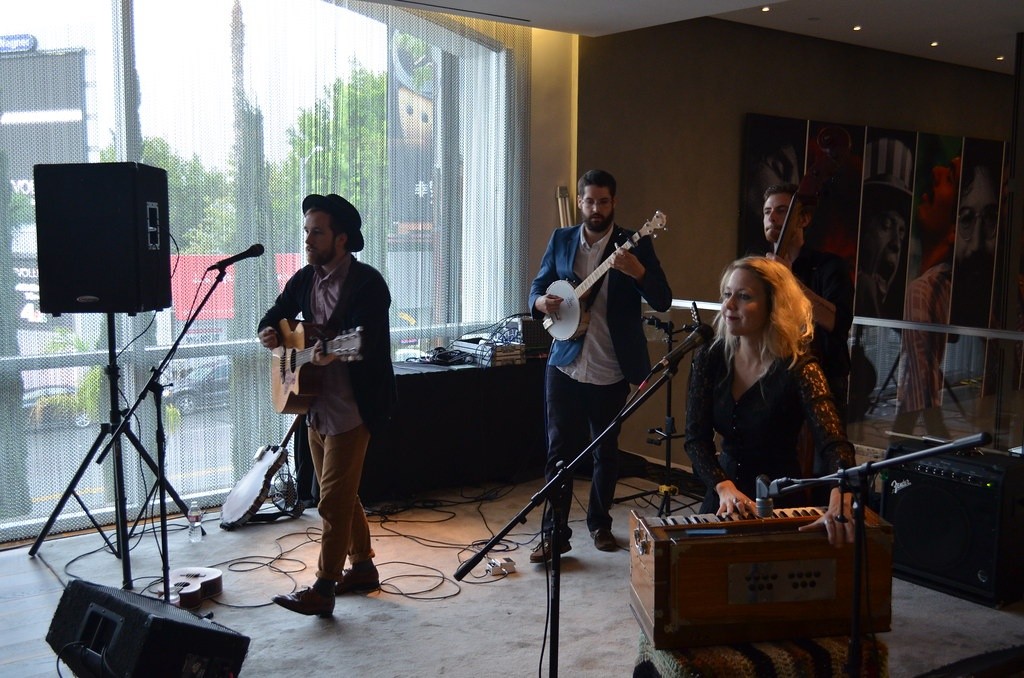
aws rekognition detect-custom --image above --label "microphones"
[756,475,774,517]
[206,244,264,271]
[650,324,715,374]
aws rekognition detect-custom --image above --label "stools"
[631,625,893,678]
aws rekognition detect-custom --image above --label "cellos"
[772,125,855,504]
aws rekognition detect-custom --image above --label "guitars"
[541,209,670,344]
[268,316,367,414]
[220,414,301,527]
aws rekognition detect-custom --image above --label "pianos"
[623,502,895,652]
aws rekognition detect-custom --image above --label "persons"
[260,194,396,620]
[822,172,997,431]
[683,183,857,549]
[527,171,672,564]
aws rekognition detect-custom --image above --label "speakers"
[44,580,251,678]
[879,440,1024,610]
[35,162,172,318]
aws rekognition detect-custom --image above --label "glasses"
[578,197,612,209]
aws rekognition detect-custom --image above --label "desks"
[292,351,590,512]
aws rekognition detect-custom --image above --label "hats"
[864,172,913,202]
[303,193,364,252]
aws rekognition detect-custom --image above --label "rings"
[733,497,741,504]
[743,499,751,504]
[824,520,833,525]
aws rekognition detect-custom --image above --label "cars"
[22,384,93,432]
[162,360,232,415]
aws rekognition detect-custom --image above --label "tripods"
[613,316,703,517]
[30,314,205,558]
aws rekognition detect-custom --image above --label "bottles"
[187,501,203,543]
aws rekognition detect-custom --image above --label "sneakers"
[590,529,617,552]
[272,586,335,618]
[530,538,572,563]
[335,566,378,596]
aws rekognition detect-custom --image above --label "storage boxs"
[450,338,528,366]
